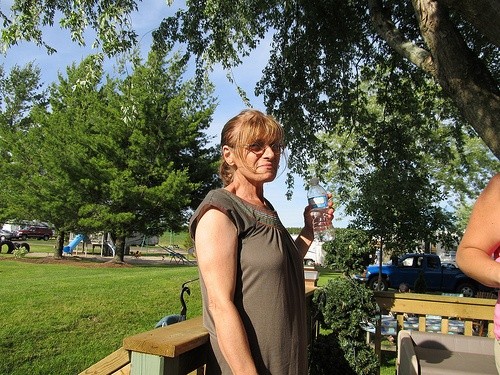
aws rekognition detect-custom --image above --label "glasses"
[245,142,286,154]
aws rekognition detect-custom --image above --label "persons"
[188,110,335,375]
[456,173,500,375]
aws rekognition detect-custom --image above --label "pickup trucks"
[365,254,473,293]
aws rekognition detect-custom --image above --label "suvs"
[15,226,54,241]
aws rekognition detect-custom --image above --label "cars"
[0,230,14,241]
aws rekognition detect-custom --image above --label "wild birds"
[155,287,191,329]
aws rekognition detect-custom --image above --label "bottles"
[307,178,335,241]
